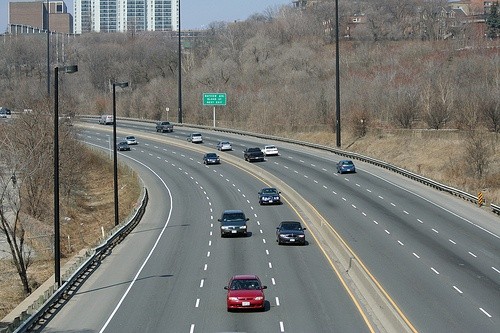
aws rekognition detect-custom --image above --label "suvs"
[0,108,11,117]
[217,210,250,237]
[187,133,203,143]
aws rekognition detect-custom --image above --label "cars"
[276,221,307,245]
[224,274,267,312]
[203,152,220,164]
[117,142,130,151]
[261,145,279,156]
[125,135,137,144]
[257,188,282,205]
[217,141,232,151]
[336,160,355,174]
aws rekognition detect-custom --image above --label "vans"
[98,115,113,124]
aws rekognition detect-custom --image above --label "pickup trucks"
[156,121,173,132]
[244,147,265,162]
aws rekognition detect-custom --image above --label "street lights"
[53,63,79,287]
[112,81,129,225]
